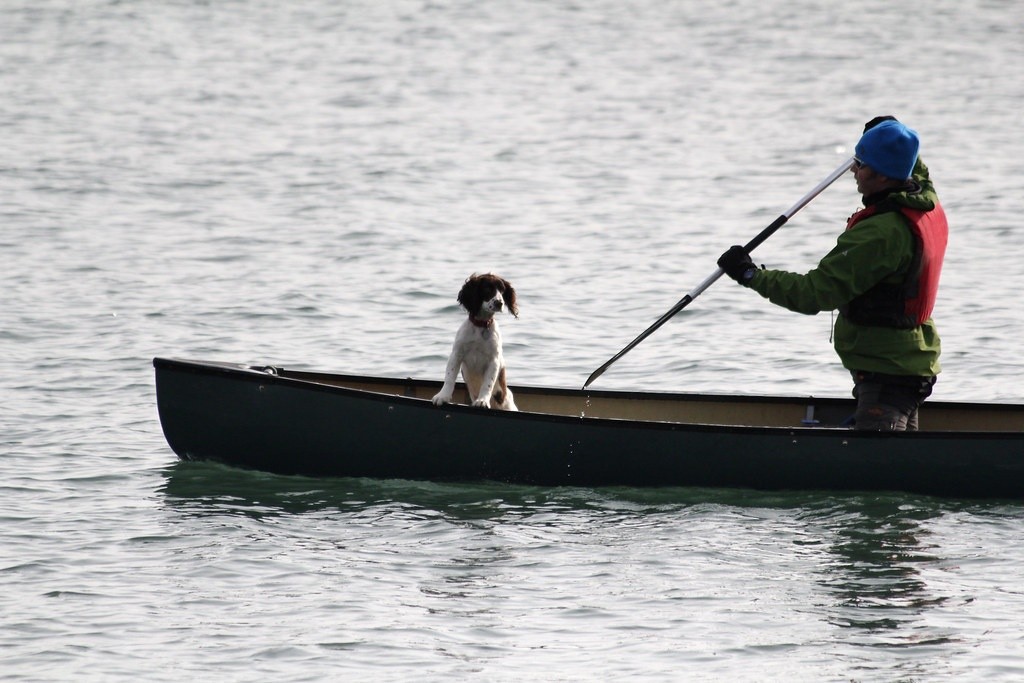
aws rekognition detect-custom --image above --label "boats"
[152,353,1023,506]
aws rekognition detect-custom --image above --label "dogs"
[430,272,519,412]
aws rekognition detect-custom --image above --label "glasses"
[852,155,865,169]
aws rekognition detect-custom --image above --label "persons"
[718,115,948,433]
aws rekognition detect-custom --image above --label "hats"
[855,120,920,182]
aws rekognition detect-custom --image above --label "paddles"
[577,157,857,388]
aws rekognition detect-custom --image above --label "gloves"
[718,246,757,287]
[863,115,897,135]
